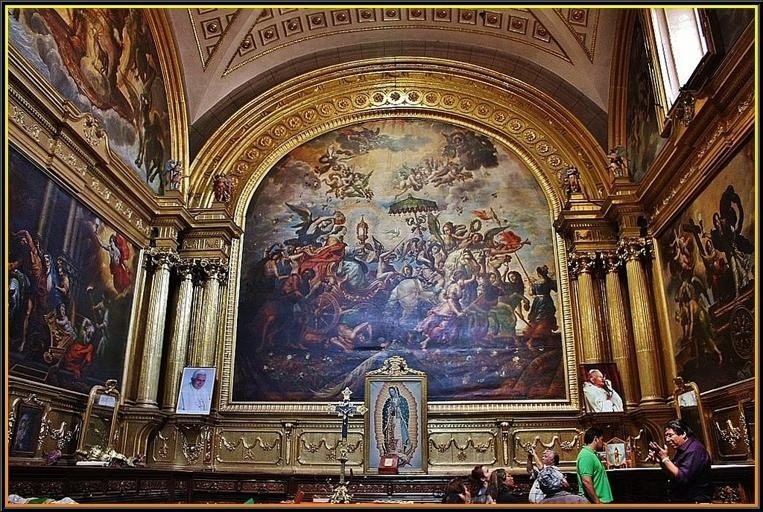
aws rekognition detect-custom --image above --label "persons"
[582,369,624,412]
[526,445,559,503]
[574,426,613,503]
[646,418,713,503]
[468,464,495,503]
[486,468,531,503]
[537,466,591,504]
[440,477,472,504]
[178,370,211,410]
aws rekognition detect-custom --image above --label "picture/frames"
[174,366,216,416]
[363,355,427,474]
[8,393,48,457]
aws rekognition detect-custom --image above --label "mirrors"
[76,379,120,451]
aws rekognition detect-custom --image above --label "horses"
[485,254,530,312]
[386,246,481,322]
